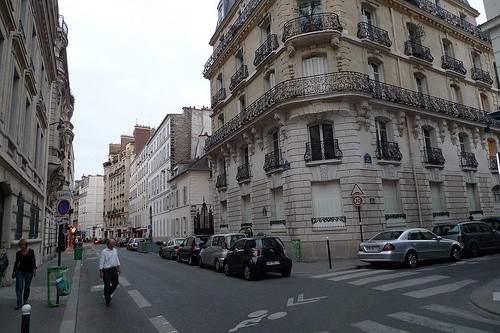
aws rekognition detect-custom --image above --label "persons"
[73,237,83,248]
[99,239,121,307]
[469,213,473,221]
[12,238,37,310]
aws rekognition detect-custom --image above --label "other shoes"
[15,305,22,310]
[106,301,112,306]
[23,300,28,304]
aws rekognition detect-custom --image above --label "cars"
[177,233,211,266]
[197,233,249,272]
[94,237,145,250]
[162,232,185,260]
[222,235,293,281]
[356,227,463,268]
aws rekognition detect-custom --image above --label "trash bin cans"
[73,247,84,261]
[47,265,71,308]
[291,238,303,262]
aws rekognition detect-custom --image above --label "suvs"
[431,221,500,258]
